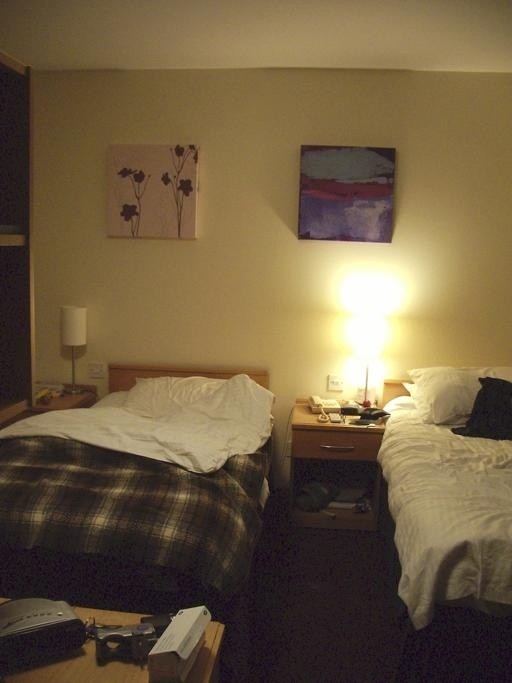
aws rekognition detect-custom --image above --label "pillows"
[403,365,512,430]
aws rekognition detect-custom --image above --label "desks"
[2,594,229,682]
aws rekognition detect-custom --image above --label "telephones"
[307,395,341,413]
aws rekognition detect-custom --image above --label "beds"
[1,359,275,625]
[372,378,511,622]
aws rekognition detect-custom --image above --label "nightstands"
[285,402,388,533]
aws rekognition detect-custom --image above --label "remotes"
[329,413,342,423]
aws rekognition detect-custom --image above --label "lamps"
[59,303,87,395]
